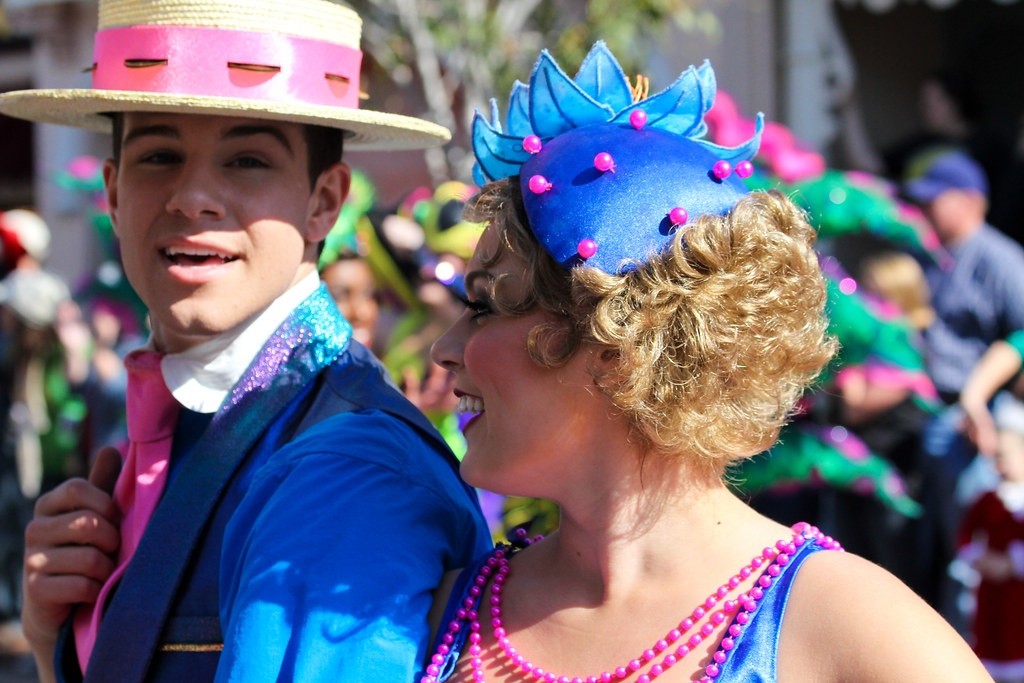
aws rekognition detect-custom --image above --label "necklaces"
[420,522,844,683]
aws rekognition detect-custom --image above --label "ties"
[73,347,179,677]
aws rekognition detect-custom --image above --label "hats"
[1,1,453,151]
[899,149,988,201]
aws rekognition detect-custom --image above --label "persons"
[826,142,1024,640]
[426,120,1024,683]
[0,109,555,683]
